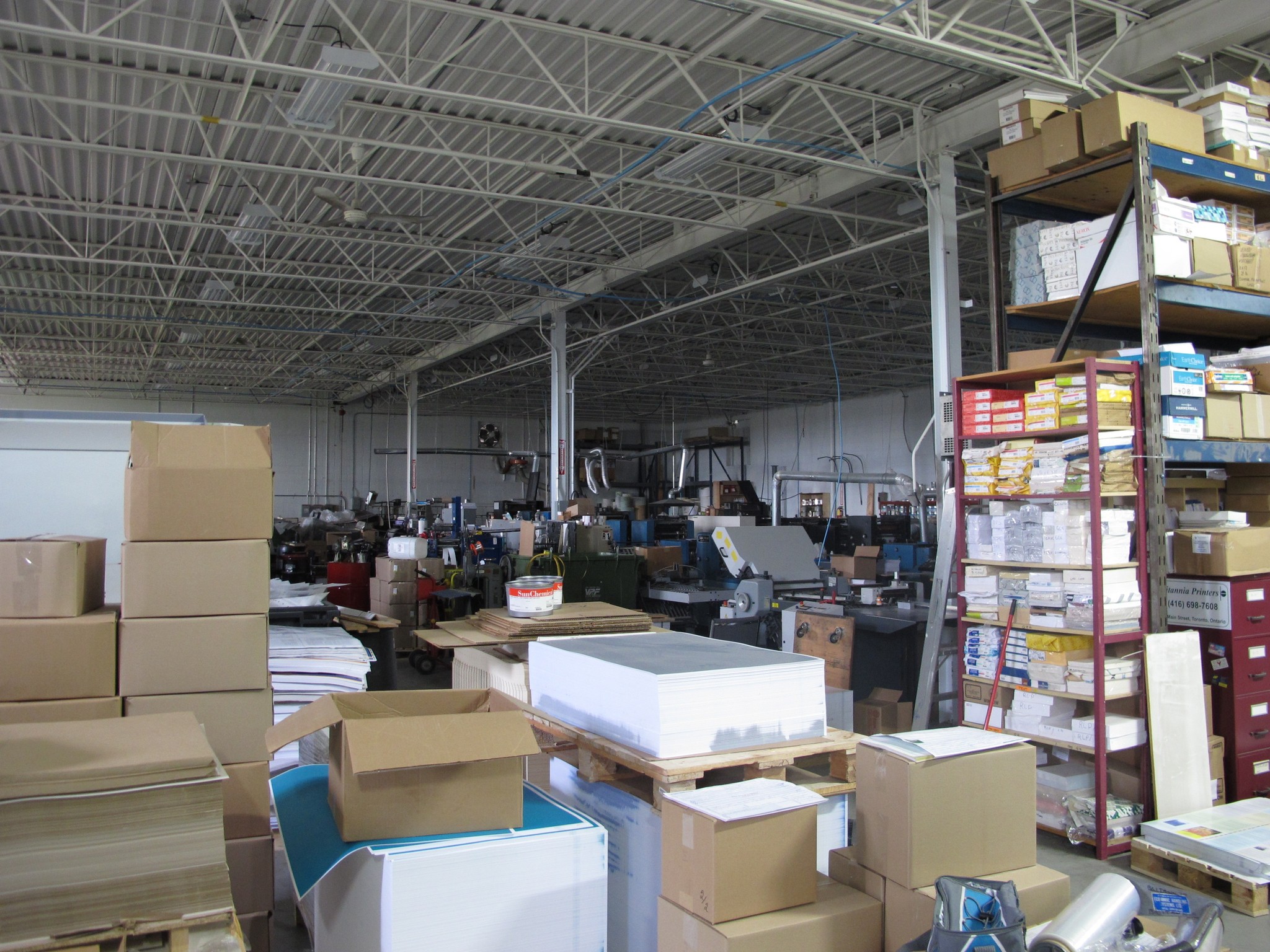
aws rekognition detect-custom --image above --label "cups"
[705,508,710,516]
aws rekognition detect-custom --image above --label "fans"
[313,146,434,227]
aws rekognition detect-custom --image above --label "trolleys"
[408,569,453,674]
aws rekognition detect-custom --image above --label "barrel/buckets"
[356,554,366,563]
[327,561,371,612]
[388,536,427,560]
[505,576,563,617]
[282,551,307,584]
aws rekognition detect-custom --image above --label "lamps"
[285,47,380,132]
[196,280,235,306]
[655,124,770,182]
[226,203,282,246]
[498,235,570,270]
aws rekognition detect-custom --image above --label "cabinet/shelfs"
[951,356,1150,859]
[971,124,1269,862]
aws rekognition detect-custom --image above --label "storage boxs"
[960,90,1270,801]
[1,424,1071,952]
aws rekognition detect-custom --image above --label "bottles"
[516,511,523,520]
[535,510,541,521]
